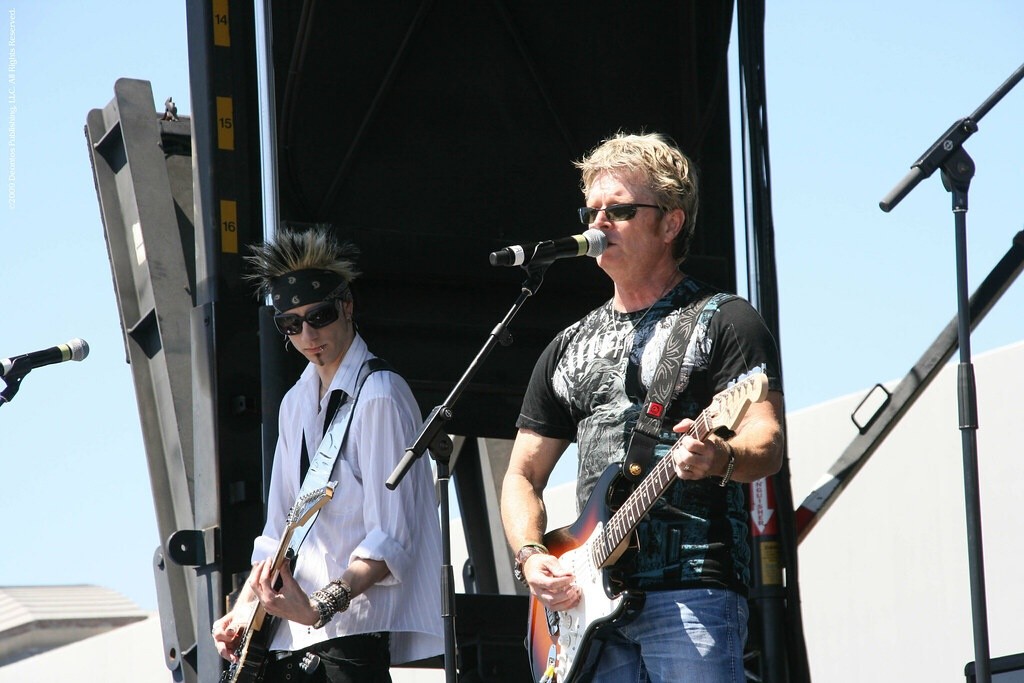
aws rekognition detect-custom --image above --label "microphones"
[0,338,89,377]
[489,228,608,266]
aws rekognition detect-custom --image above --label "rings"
[211,625,221,635]
[683,463,690,472]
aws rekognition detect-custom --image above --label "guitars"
[226,477,339,683]
[526,361,769,683]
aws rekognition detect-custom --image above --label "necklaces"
[612,270,677,359]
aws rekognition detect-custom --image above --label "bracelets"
[716,435,735,486]
[514,542,550,588]
[307,579,352,630]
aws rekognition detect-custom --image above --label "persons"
[500,133,784,683]
[212,228,446,683]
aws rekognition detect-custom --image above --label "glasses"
[273,298,339,335]
[576,202,667,223]
